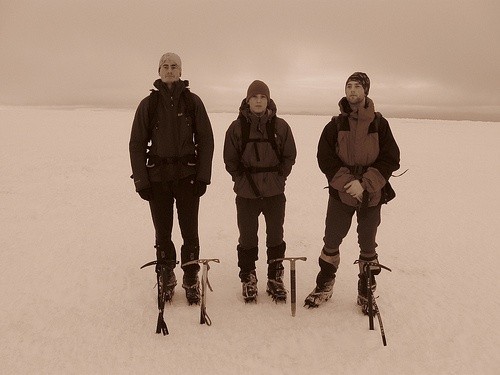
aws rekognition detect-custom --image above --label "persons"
[224,80,298,302]
[129,53,214,306]
[303,72,401,318]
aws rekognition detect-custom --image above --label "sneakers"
[241,273,259,304]
[156,268,178,303]
[266,275,288,304]
[356,272,377,316]
[182,269,201,306]
[303,277,335,309]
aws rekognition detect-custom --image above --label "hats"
[245,79,270,102]
[345,72,371,94]
[159,52,182,68]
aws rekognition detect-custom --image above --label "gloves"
[343,179,365,203]
[138,187,152,201]
[192,181,207,197]
